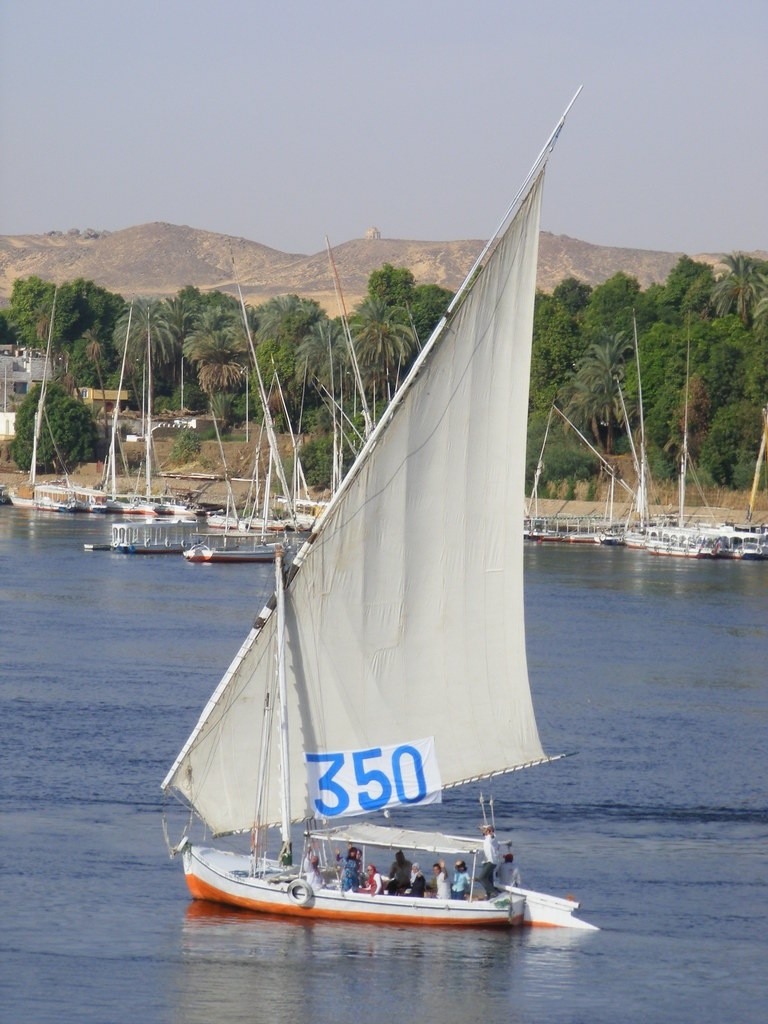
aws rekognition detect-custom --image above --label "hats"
[455,860,467,871]
[503,853,513,860]
[483,824,495,832]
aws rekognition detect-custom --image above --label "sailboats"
[0,236,319,562]
[136,81,597,935]
[522,305,768,559]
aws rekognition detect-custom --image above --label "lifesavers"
[600,534,606,541]
[287,879,312,904]
[618,537,623,542]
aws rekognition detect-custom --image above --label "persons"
[401,863,426,898]
[335,846,384,896]
[496,854,520,887]
[432,859,451,899]
[304,844,337,890]
[451,860,470,900]
[479,824,498,901]
[388,850,412,896]
